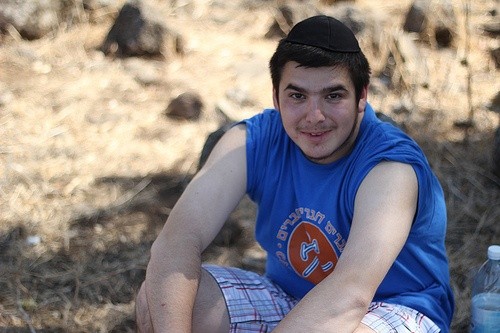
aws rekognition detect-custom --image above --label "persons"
[135,16,456,333]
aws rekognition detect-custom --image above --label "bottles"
[467,244,500,332]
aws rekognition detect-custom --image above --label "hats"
[281,15,361,54]
